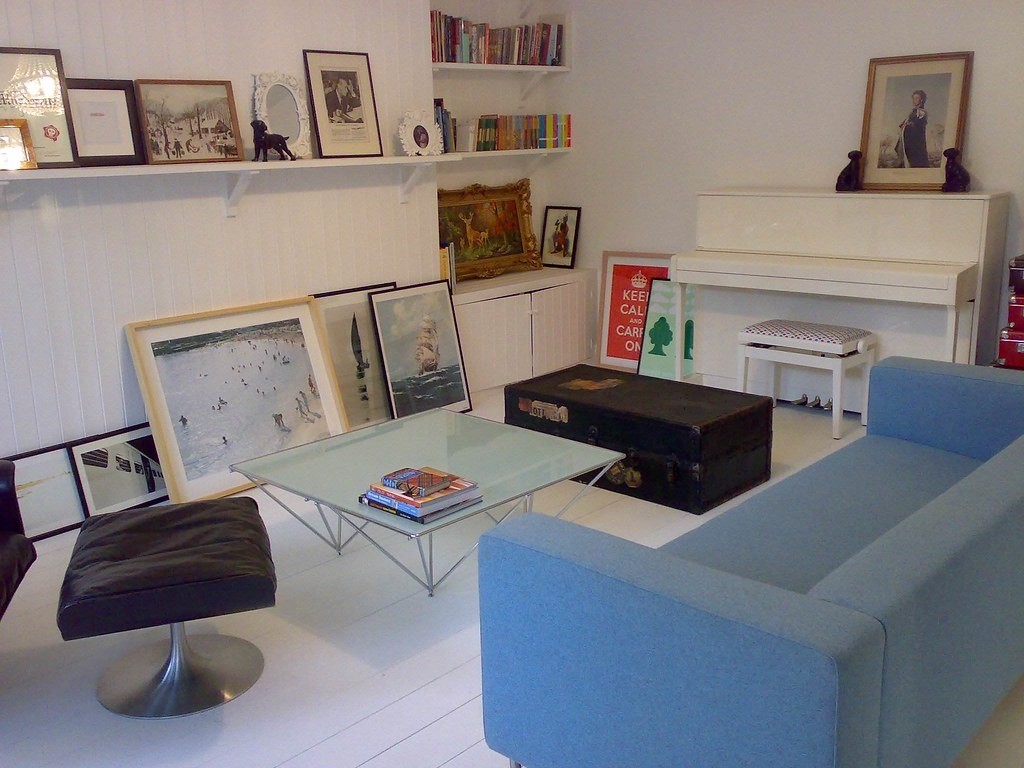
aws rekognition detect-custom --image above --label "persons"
[325,76,361,117]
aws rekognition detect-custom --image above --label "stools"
[737,319,875,439]
[57,496,275,718]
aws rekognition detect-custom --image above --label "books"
[436,97,571,154]
[430,9,563,66]
[359,466,483,524]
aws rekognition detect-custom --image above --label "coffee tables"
[231,406,626,597]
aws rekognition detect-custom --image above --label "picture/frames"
[0,118,39,170]
[124,295,353,503]
[599,249,672,371]
[303,49,385,157]
[539,205,582,270]
[369,280,472,421]
[67,424,170,518]
[397,108,444,155]
[0,46,81,169]
[65,78,146,168]
[0,442,85,543]
[308,282,396,432]
[858,50,974,191]
[438,178,543,279]
[636,278,693,383]
[135,78,243,165]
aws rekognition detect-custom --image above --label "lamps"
[252,71,312,159]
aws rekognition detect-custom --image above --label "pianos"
[668,189,1012,416]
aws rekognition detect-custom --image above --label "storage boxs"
[1007,254,1024,294]
[1008,295,1024,329]
[998,326,1024,369]
[509,367,772,515]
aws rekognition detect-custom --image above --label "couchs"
[478,357,1024,768]
[0,460,37,620]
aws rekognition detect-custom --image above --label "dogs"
[941,148,971,192]
[250,120,297,162]
[836,150,862,193]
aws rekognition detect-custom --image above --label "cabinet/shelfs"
[434,60,572,160]
[451,270,598,398]
